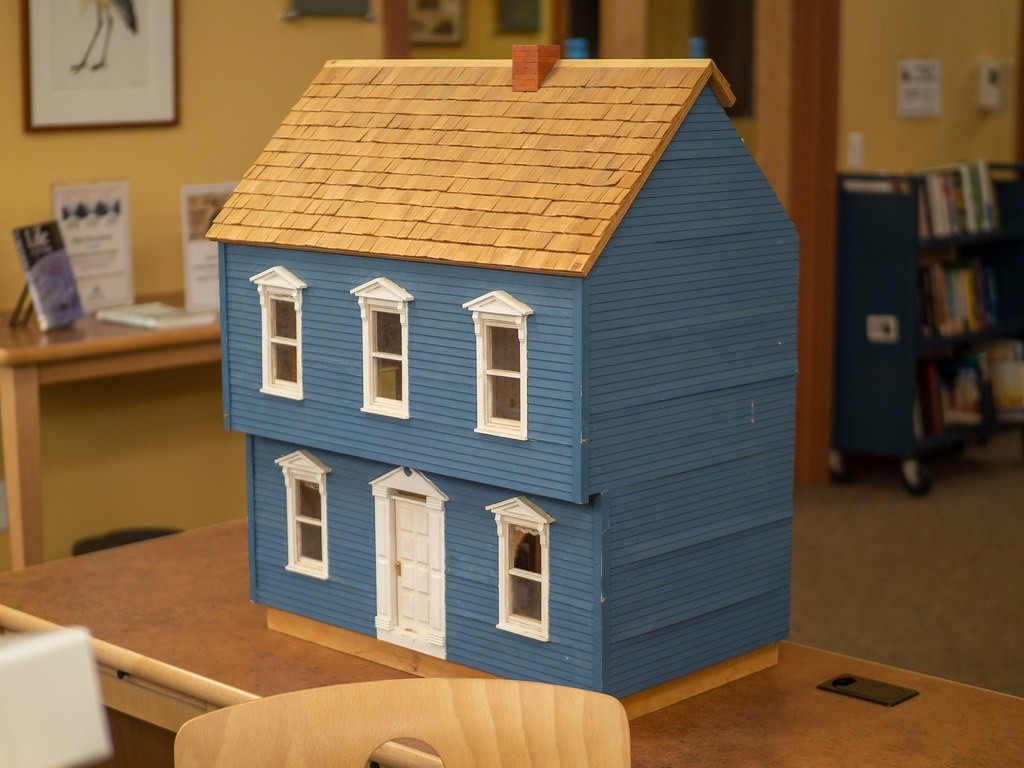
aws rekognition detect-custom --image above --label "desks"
[0,516,1024,768]
[0,288,229,570]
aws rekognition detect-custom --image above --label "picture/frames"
[21,0,180,132]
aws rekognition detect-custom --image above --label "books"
[890,160,999,241]
[940,351,996,425]
[974,339,1024,414]
[95,301,218,329]
[13,221,85,332]
[920,252,997,337]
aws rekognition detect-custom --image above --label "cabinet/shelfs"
[829,162,1024,497]
